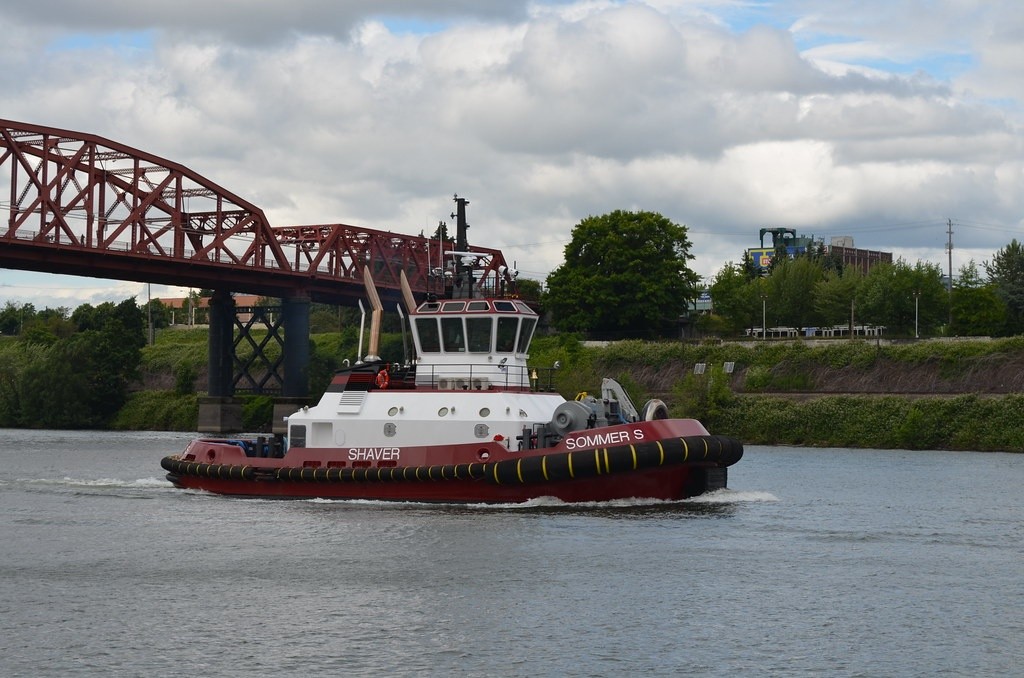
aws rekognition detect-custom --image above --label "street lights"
[913,292,921,338]
[760,295,768,339]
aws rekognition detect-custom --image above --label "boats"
[161,193,744,505]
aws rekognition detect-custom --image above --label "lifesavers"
[375,369,390,389]
